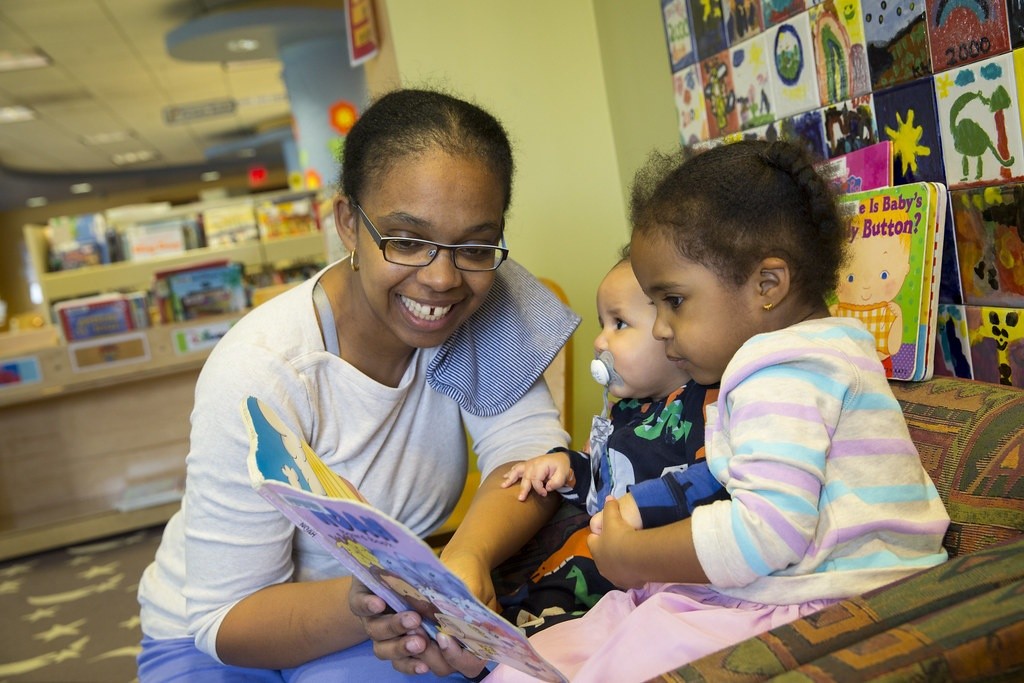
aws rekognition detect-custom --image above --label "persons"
[493,245,732,639]
[137,89,570,683]
[480,139,949,683]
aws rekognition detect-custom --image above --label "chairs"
[423,277,575,540]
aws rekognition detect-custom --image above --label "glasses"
[349,195,509,272]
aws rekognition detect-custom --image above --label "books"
[240,394,571,683]
[822,181,948,383]
[45,189,321,345]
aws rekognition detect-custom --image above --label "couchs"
[641,370,1024,683]
